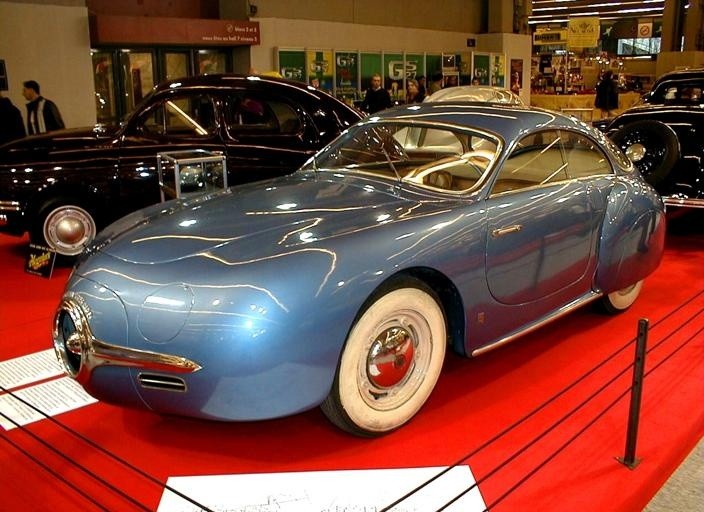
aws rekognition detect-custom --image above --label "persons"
[594,69,618,117]
[511,73,521,92]
[22,80,64,133]
[359,72,482,117]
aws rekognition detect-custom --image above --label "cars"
[1,73,412,267]
[419,86,524,107]
[590,68,704,236]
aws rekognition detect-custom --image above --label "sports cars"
[53,102,666,440]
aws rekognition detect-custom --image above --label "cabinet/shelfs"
[155,147,229,206]
